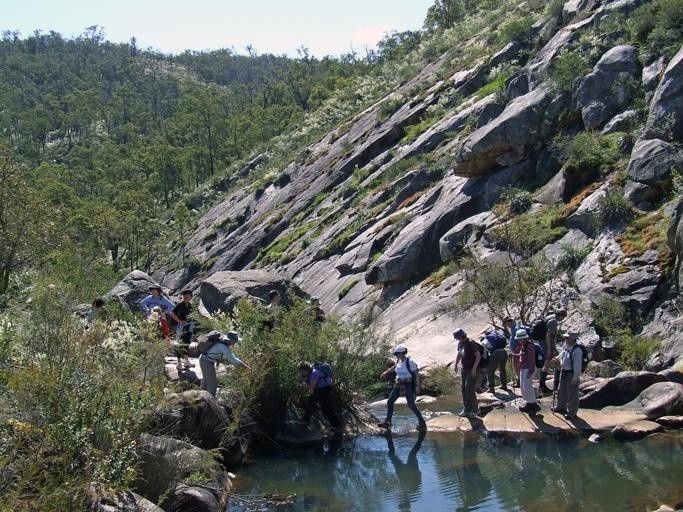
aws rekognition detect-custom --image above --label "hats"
[148,283,161,290]
[563,330,578,339]
[454,329,465,339]
[227,331,238,340]
[502,317,513,326]
[556,309,567,316]
[182,290,192,295]
[393,346,407,354]
[514,329,529,339]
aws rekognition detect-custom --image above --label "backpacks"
[569,344,589,373]
[529,316,554,340]
[196,333,228,356]
[310,361,333,379]
[524,341,544,368]
[485,329,506,349]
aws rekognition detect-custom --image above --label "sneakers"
[176,364,185,370]
[499,385,507,389]
[378,422,390,428]
[469,409,480,419]
[565,412,576,418]
[184,361,195,367]
[458,409,473,416]
[520,403,540,413]
[416,420,426,430]
[487,389,495,393]
[296,420,307,425]
[551,406,563,412]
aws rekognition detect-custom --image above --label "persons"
[508,329,541,412]
[499,314,531,387]
[375,344,428,431]
[86,285,327,399]
[481,330,508,393]
[550,329,584,420]
[295,359,342,426]
[451,328,482,419]
[539,308,561,392]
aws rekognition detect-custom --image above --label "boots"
[539,370,560,393]
[512,378,520,388]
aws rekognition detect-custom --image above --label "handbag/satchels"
[470,339,489,367]
